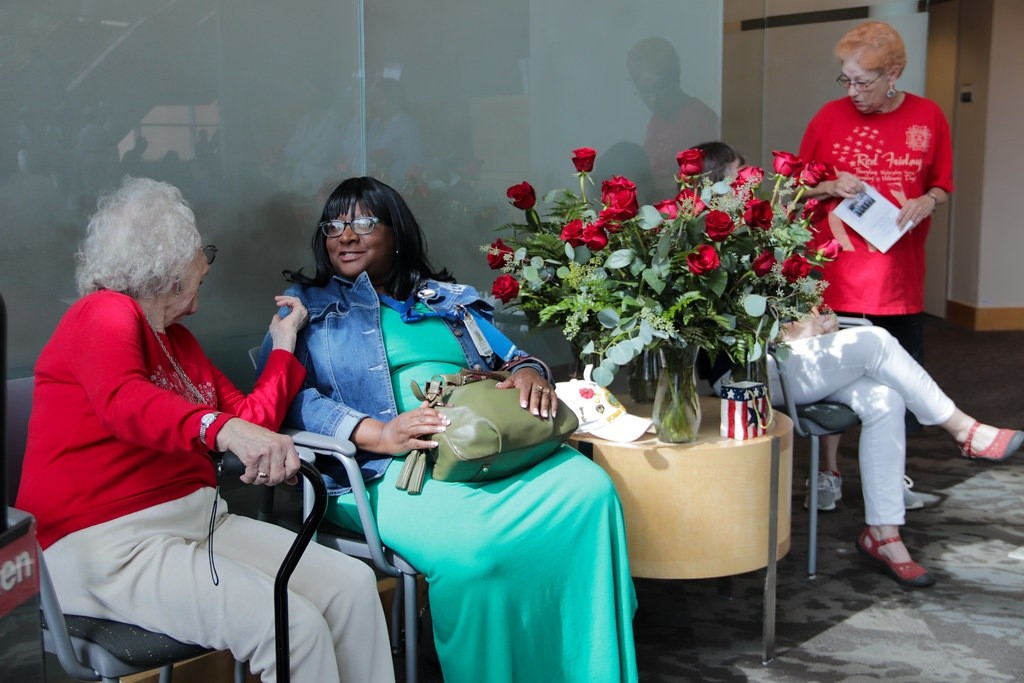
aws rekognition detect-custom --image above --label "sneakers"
[805,470,842,510]
[903,475,924,510]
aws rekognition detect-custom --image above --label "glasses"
[319,217,382,238]
[195,245,217,265]
[836,74,880,91]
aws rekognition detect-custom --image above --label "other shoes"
[855,528,936,588]
[958,424,1024,462]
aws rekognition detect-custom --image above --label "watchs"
[199,411,223,445]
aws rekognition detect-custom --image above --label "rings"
[537,384,551,394]
[257,471,270,478]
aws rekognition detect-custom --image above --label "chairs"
[706,315,874,581]
[5,346,422,683]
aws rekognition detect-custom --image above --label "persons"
[119,130,222,173]
[794,20,954,510]
[258,175,638,683]
[677,143,1024,587]
[15,178,395,683]
[627,37,722,199]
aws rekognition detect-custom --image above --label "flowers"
[477,147,844,439]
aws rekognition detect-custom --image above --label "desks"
[542,397,794,665]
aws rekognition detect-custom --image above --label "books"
[832,181,914,254]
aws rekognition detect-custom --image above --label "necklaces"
[139,307,209,405]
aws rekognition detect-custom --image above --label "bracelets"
[928,191,937,212]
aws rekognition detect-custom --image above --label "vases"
[653,342,702,443]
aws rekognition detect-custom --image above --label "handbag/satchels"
[203,452,304,586]
[395,362,580,494]
